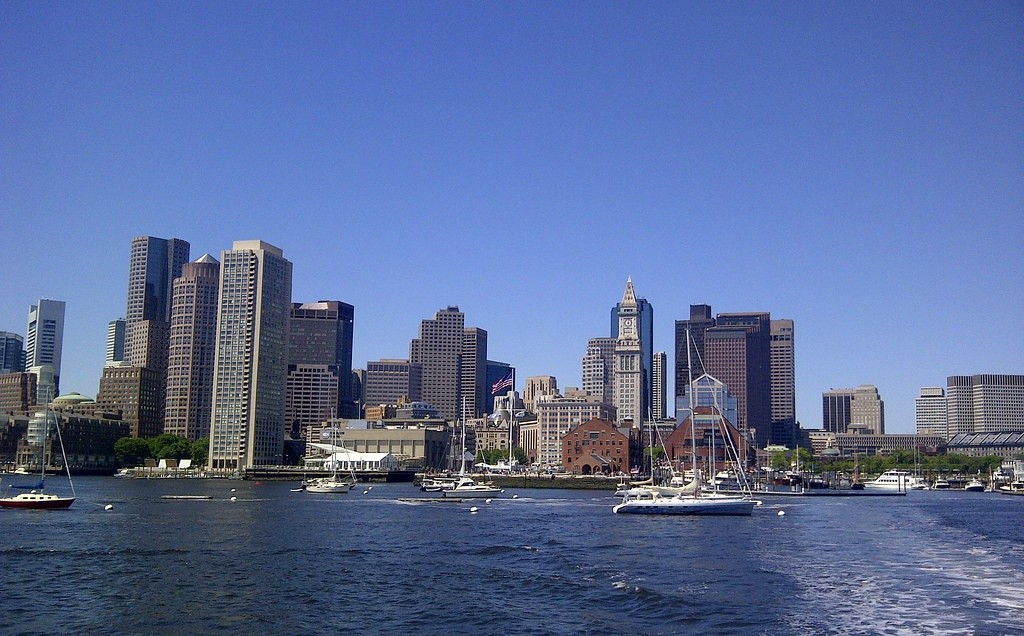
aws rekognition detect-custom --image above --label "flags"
[490,371,512,394]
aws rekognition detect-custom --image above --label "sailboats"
[612,328,755,516]
[0,386,76,510]
[301,406,358,493]
[415,397,502,498]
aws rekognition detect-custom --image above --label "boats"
[1000,482,1024,491]
[931,479,950,488]
[965,479,984,492]
[863,470,927,491]
